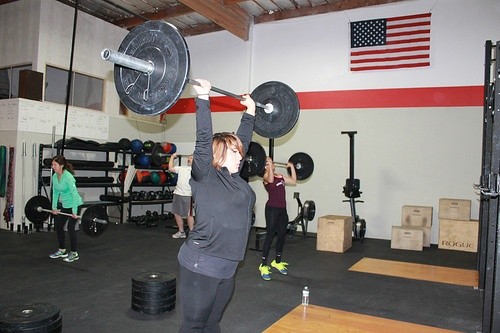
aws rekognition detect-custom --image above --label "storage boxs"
[438,198,479,252]
[316,215,352,253]
[391,205,432,251]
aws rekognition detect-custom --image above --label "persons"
[168,153,195,238]
[258,157,297,281]
[178,80,256,333]
[49,156,83,263]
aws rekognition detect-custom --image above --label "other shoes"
[172,231,186,238]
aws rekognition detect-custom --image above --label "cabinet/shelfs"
[37,143,181,234]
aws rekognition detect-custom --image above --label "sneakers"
[63,251,79,262]
[271,259,289,275]
[49,249,68,258]
[258,263,272,280]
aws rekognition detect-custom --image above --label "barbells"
[99,19,300,139]
[240,142,315,180]
[25,196,109,237]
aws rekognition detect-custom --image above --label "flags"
[350,13,431,71]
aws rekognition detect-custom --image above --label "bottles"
[302,286,309,306]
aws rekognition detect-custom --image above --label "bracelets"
[197,93,209,96]
